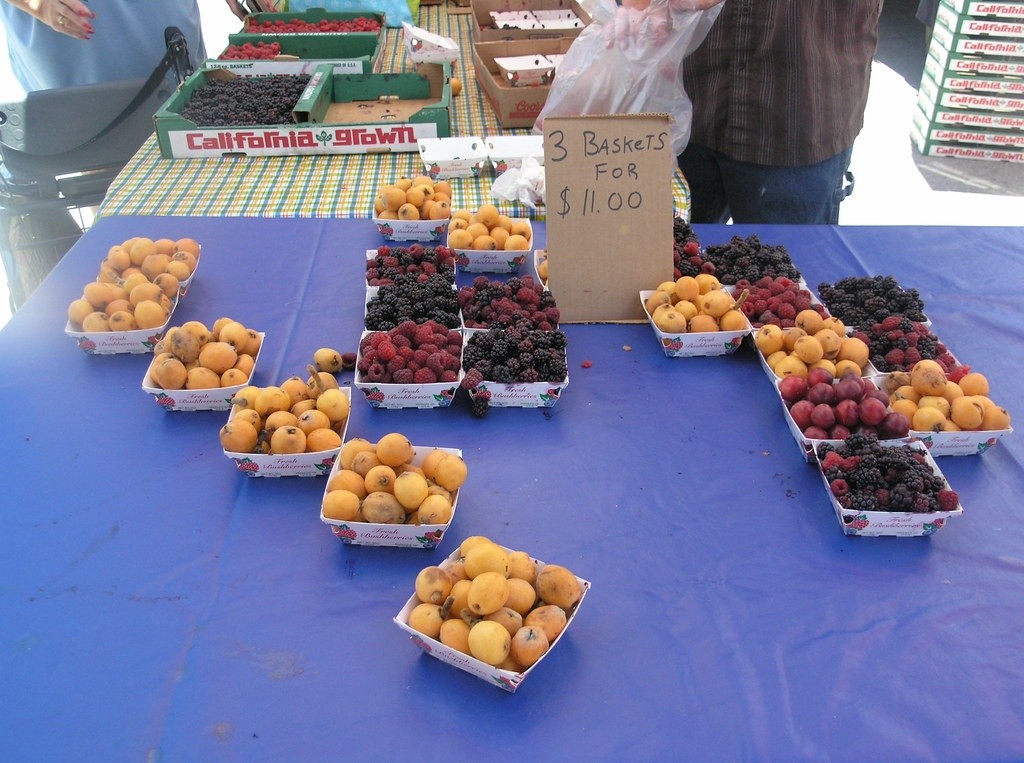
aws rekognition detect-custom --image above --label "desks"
[0,216,1023,763]
[90,1,691,223]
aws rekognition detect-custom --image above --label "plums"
[779,368,910,441]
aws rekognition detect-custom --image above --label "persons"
[604,0,882,226]
[0,1,203,96]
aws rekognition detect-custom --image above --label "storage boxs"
[151,1,591,159]
[909,0,1024,166]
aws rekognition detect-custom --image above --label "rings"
[56,15,65,26]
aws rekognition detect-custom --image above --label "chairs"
[0,74,177,316]
